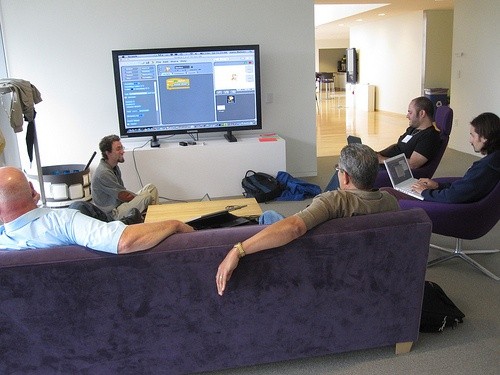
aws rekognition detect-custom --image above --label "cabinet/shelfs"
[353,85,375,110]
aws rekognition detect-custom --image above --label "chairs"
[319,73,335,97]
[398,175,500,287]
[375,104,453,188]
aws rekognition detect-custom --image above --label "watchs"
[233,241,245,257]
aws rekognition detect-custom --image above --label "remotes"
[188,141,196,145]
[179,142,187,146]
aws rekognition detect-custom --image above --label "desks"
[143,197,263,222]
[119,131,286,201]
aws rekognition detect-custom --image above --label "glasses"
[334,164,346,173]
[116,147,125,151]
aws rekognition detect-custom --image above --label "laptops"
[384,153,430,200]
[347,135,362,145]
[185,204,250,231]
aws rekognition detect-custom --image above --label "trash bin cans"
[426,88,449,121]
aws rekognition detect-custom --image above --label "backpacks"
[241,170,281,202]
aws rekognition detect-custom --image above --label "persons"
[323,97,441,193]
[91,134,158,220]
[215,143,400,296]
[379,112,500,203]
[0,166,194,254]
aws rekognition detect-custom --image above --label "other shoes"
[120,208,143,225]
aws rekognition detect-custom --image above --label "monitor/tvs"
[111,45,262,147]
[346,48,358,83]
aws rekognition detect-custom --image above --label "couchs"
[0,205,431,375]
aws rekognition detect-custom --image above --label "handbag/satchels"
[419,280,465,332]
